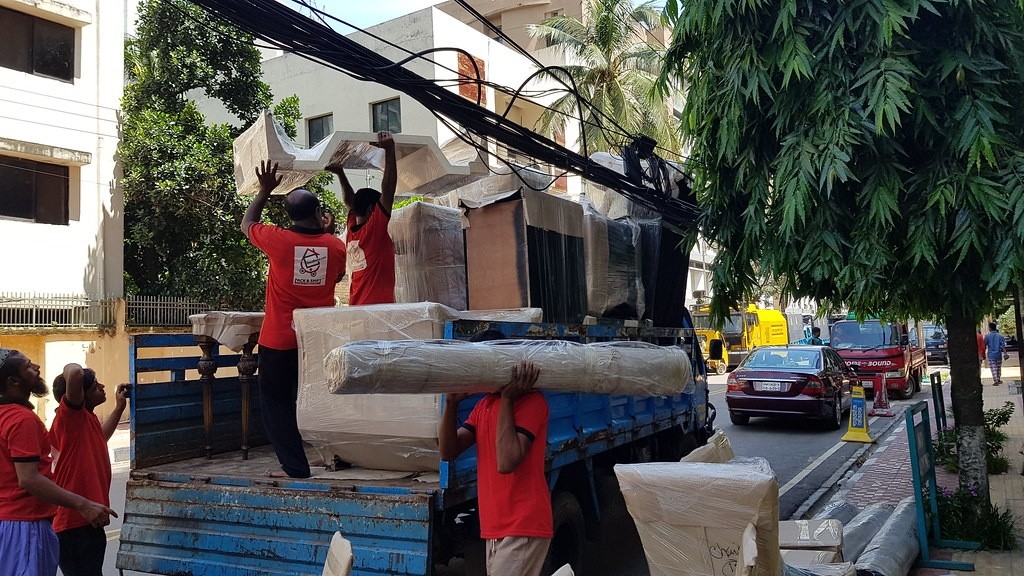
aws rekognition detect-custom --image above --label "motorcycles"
[696,330,728,374]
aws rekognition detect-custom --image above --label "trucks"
[782,313,814,346]
[113,304,717,576]
[829,317,927,401]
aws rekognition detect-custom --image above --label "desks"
[188,309,264,462]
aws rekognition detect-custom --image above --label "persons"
[241,158,345,477]
[984,323,1009,386]
[834,325,849,344]
[439,329,554,576]
[324,130,398,305]
[0,348,118,576]
[807,328,822,364]
[977,331,985,378]
[48,363,130,576]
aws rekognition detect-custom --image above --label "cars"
[726,343,863,430]
[911,325,950,365]
[1000,337,1018,352]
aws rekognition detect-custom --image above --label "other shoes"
[262,469,309,480]
[993,379,1003,386]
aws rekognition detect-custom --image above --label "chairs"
[609,429,858,575]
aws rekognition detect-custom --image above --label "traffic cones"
[867,373,896,417]
[841,387,878,443]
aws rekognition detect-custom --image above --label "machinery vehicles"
[689,303,789,367]
